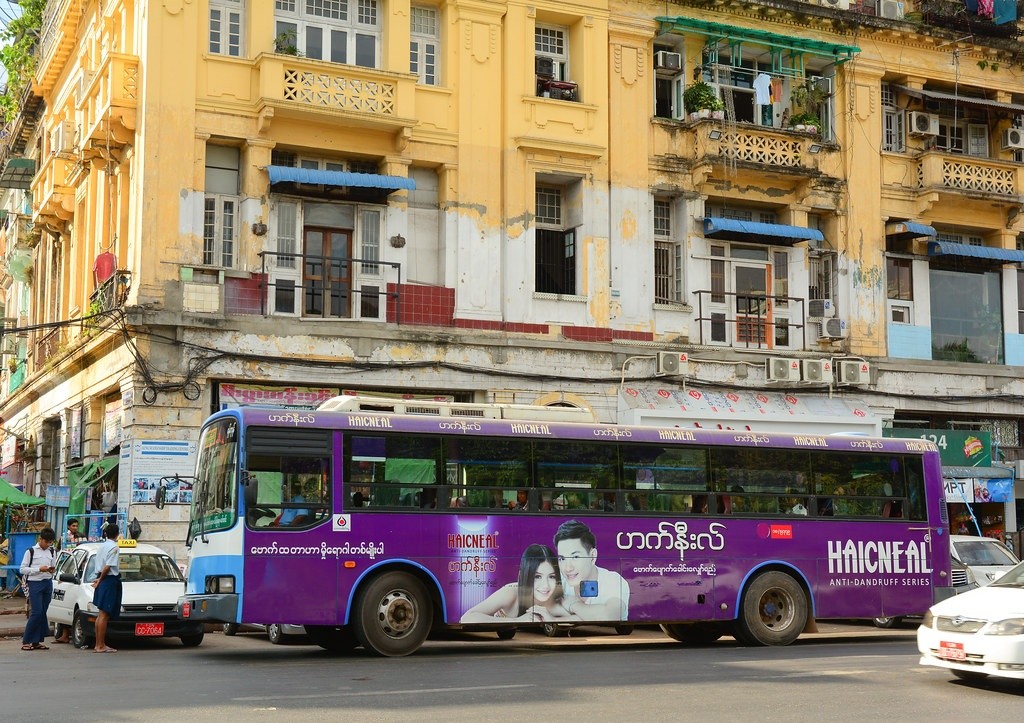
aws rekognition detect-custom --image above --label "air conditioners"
[656,351,688,374]
[908,111,940,136]
[765,358,799,381]
[877,0,903,19]
[16,315,28,337]
[654,50,681,69]
[836,361,870,385]
[800,359,833,384]
[50,121,74,152]
[817,318,846,338]
[808,298,833,318]
[0,334,16,355]
[1001,127,1024,150]
[819,0,849,10]
[807,75,832,94]
[5,213,18,233]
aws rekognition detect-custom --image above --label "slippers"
[21,645,33,651]
[50,638,70,643]
[94,646,116,653]
[33,644,49,650]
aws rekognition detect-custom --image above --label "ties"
[274,513,283,527]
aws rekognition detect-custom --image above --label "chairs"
[690,495,732,514]
[142,565,159,580]
[627,493,648,510]
[451,496,467,508]
[804,498,834,516]
[536,56,578,101]
[466,490,503,508]
[374,479,401,507]
[419,479,453,509]
[540,487,551,511]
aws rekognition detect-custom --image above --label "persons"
[274,479,308,526]
[19,528,55,650]
[701,484,886,515]
[350,478,375,508]
[91,523,122,653]
[458,520,630,623]
[451,487,529,509]
[52,518,86,644]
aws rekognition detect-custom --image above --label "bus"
[154,392,955,662]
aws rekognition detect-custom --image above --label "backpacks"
[21,547,53,597]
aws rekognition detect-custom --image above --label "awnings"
[262,164,416,191]
[943,464,1014,479]
[885,222,938,239]
[658,16,862,76]
[704,216,826,248]
[928,241,1024,263]
[0,157,36,189]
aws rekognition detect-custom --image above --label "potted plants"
[273,26,307,100]
[683,81,724,120]
[789,112,821,135]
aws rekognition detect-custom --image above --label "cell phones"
[579,582,598,597]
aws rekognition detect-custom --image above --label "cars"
[916,559,1024,682]
[47,539,205,649]
[871,534,1024,629]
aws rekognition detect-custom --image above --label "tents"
[1,477,46,508]
